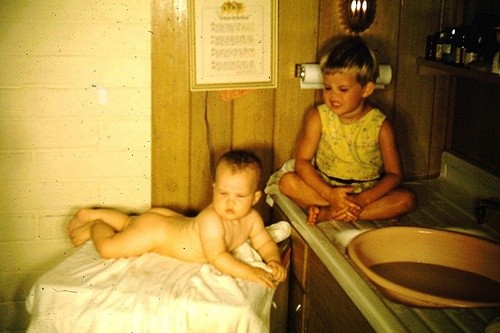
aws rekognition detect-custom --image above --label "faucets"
[474,198,500,225]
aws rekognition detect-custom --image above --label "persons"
[278,37,419,226]
[66,150,288,289]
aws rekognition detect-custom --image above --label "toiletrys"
[428,27,478,68]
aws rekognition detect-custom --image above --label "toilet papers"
[298,63,393,90]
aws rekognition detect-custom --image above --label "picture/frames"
[187,0,279,92]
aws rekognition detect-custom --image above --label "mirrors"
[338,0,377,34]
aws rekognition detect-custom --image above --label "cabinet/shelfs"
[25,243,291,332]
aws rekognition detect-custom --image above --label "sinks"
[335,228,500,309]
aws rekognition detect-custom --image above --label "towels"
[23,220,292,333]
